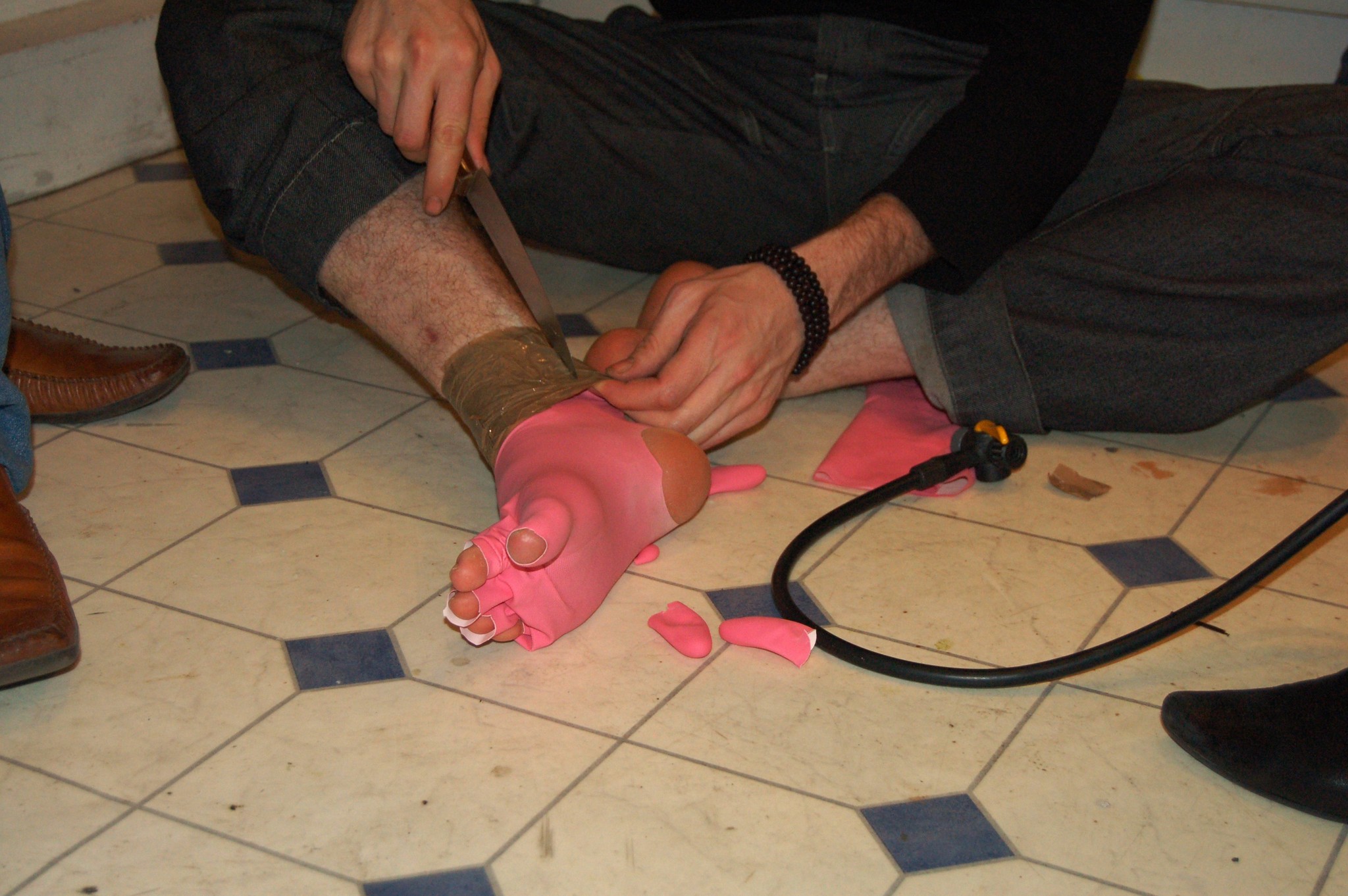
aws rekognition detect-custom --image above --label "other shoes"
[1161,667,1348,825]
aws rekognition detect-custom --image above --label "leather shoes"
[0,463,79,686]
[1,316,190,424]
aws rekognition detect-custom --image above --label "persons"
[150,0,1348,652]
[0,196,190,686]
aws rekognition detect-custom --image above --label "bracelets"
[745,244,831,375]
